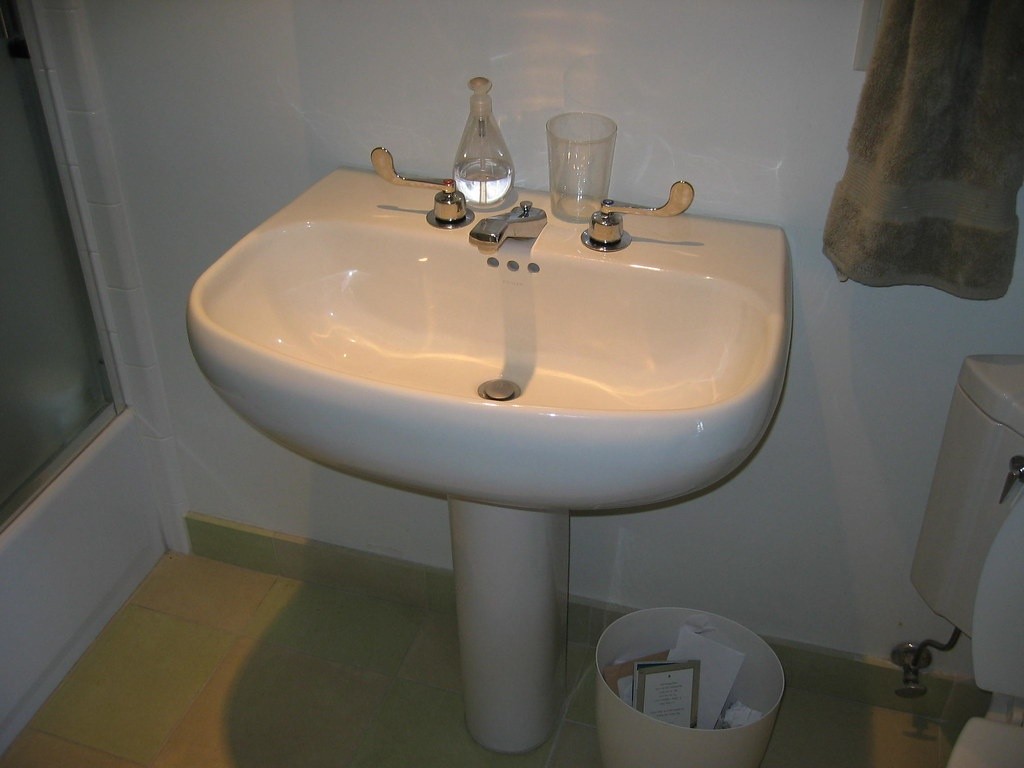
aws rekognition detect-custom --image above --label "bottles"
[452,78,515,209]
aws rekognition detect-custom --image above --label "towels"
[821,0,1024,301]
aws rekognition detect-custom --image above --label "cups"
[545,112,618,224]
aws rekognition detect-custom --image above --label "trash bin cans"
[595,606,785,768]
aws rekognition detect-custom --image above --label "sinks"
[184,217,794,515]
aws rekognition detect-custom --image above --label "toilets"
[905,347,1024,768]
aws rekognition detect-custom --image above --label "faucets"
[468,199,547,254]
